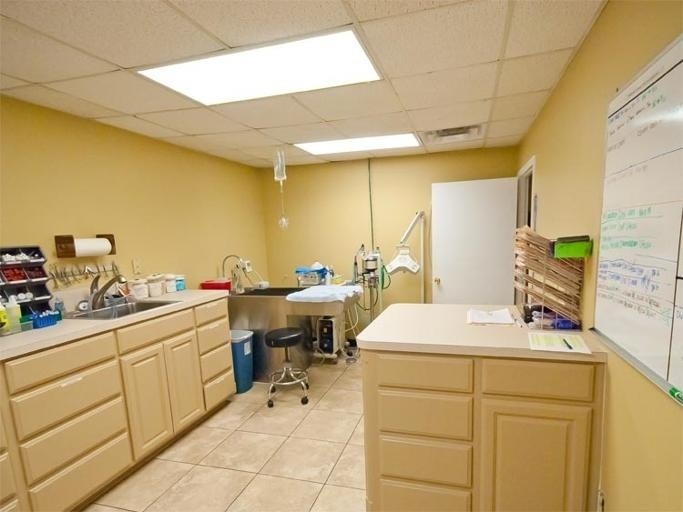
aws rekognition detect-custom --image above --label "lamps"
[386,210,427,304]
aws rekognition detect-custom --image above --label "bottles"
[0,295,6,330]
[6,295,20,331]
[53,293,64,320]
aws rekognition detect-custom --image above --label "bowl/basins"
[523,303,550,318]
[531,317,552,325]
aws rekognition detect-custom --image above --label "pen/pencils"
[512,314,523,329]
[563,339,572,349]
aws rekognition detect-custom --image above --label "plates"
[553,319,576,328]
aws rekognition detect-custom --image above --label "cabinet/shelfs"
[361,350,477,512]
[194,297,237,416]
[477,358,606,512]
[115,291,206,466]
[0,330,135,512]
[0,245,53,336]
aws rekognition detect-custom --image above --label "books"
[512,224,584,325]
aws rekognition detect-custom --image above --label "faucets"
[88,274,126,312]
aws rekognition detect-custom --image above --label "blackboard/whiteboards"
[587,34,683,409]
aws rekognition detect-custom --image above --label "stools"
[263,327,312,408]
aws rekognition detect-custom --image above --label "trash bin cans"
[230,329,256,394]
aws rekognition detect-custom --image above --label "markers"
[668,387,683,405]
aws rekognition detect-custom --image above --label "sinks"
[62,299,182,321]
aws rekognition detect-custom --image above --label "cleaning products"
[5,295,21,333]
